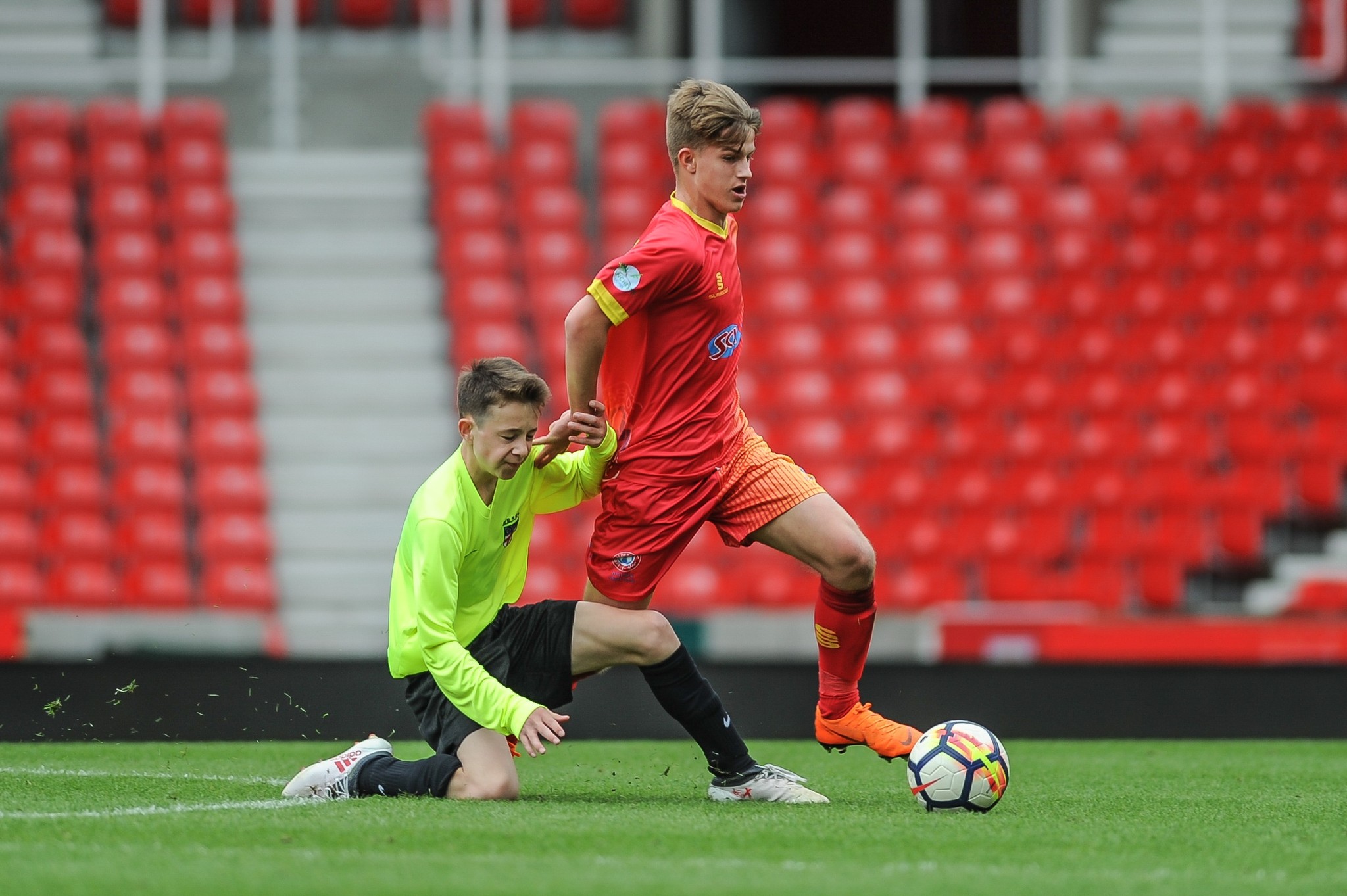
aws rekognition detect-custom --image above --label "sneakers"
[707,764,832,804]
[815,701,925,763]
[507,734,520,757]
[282,733,394,798]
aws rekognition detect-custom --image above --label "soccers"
[907,720,1010,814]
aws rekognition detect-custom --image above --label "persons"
[278,356,831,807]
[530,74,926,760]
